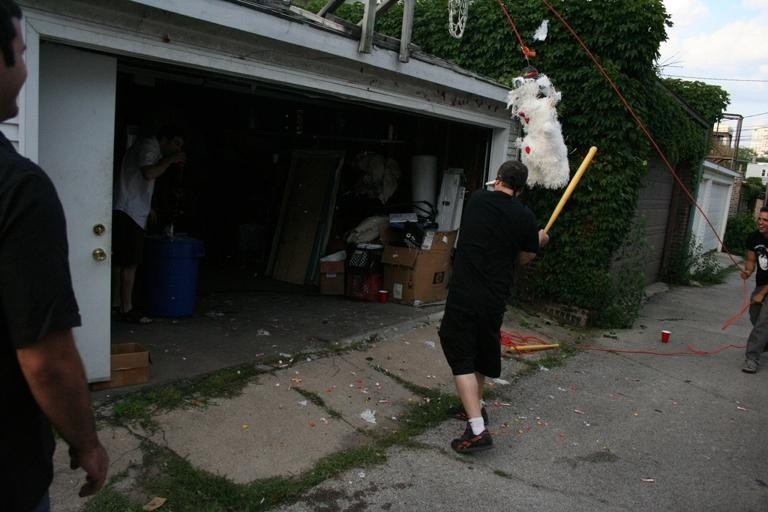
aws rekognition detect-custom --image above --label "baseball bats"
[543,146,598,234]
[507,344,559,352]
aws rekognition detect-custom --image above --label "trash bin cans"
[151,239,206,318]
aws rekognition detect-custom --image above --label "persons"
[112,136,187,325]
[740,207,768,374]
[0,0,109,512]
[438,160,550,454]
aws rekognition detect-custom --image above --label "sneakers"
[450,422,493,453]
[447,403,488,425]
[741,359,759,373]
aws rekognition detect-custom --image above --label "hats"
[496,161,528,192]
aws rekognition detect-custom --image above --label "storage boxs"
[91,342,153,390]
[380,229,461,305]
[320,250,347,295]
